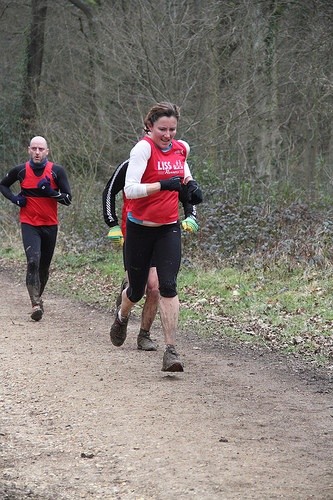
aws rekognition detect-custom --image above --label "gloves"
[181,217,198,233]
[11,190,27,208]
[37,175,54,196]
[186,180,202,205]
[107,226,124,250]
[158,176,183,192]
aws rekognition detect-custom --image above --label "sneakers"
[136,328,157,351]
[116,278,129,309]
[109,308,128,347]
[160,344,184,372]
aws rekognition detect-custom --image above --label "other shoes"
[30,300,43,321]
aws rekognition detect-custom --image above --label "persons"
[0,136,72,322]
[102,112,199,351]
[110,102,203,372]
